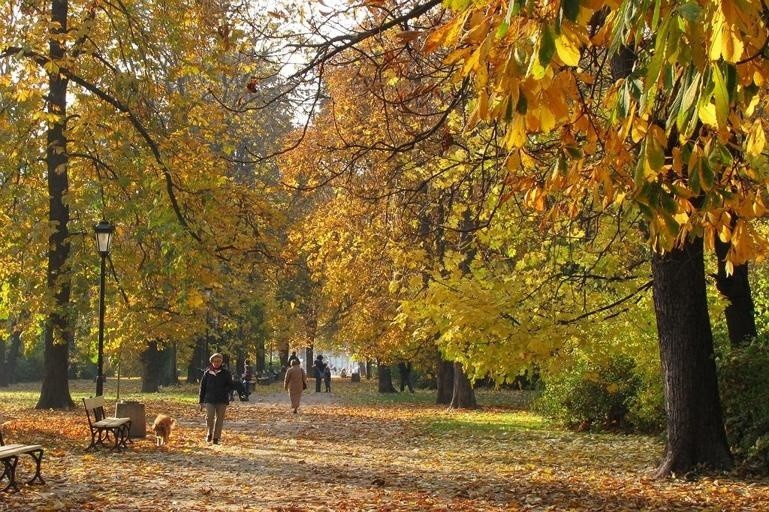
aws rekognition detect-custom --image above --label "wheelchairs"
[228,380,251,401]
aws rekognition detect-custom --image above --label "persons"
[265,351,299,384]
[198,353,233,445]
[283,360,307,414]
[313,354,352,392]
[222,359,252,402]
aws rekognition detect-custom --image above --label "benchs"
[83,396,133,452]
[256,369,281,385]
[0,434,44,492]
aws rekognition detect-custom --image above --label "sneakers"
[207,434,218,444]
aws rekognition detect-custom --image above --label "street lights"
[204,287,213,367]
[95,219,114,421]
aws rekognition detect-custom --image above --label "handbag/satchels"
[303,381,306,389]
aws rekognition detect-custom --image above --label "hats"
[210,353,223,363]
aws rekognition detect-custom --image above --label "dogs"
[151,412,176,448]
